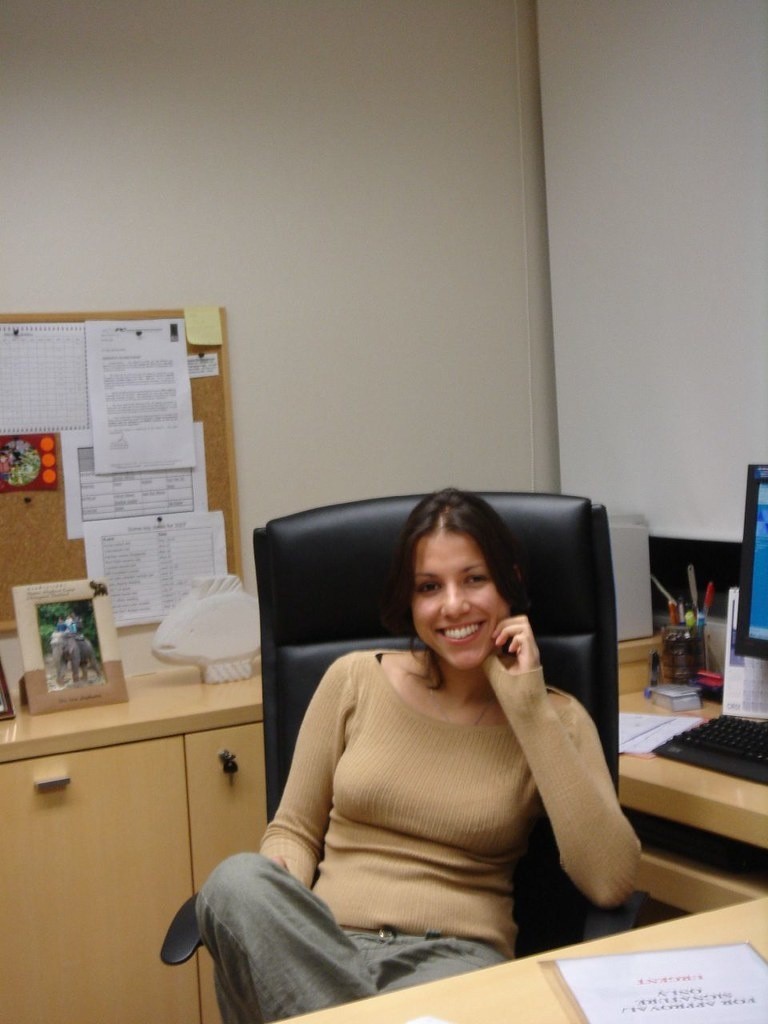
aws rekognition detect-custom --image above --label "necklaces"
[427,650,497,727]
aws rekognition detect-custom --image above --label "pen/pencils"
[667,579,714,639]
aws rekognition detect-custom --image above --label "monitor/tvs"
[735,463,768,661]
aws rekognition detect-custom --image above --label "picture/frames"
[12,580,130,712]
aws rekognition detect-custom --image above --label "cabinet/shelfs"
[0,665,264,1024]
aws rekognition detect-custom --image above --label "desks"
[266,629,768,1023]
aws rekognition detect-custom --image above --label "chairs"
[161,490,621,968]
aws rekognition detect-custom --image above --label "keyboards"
[651,714,768,785]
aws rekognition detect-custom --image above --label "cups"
[659,623,708,688]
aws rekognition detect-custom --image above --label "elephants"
[50,631,101,686]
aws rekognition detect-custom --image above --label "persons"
[196,487,641,1024]
[65,616,77,633]
[70,611,82,630]
[57,617,67,632]
